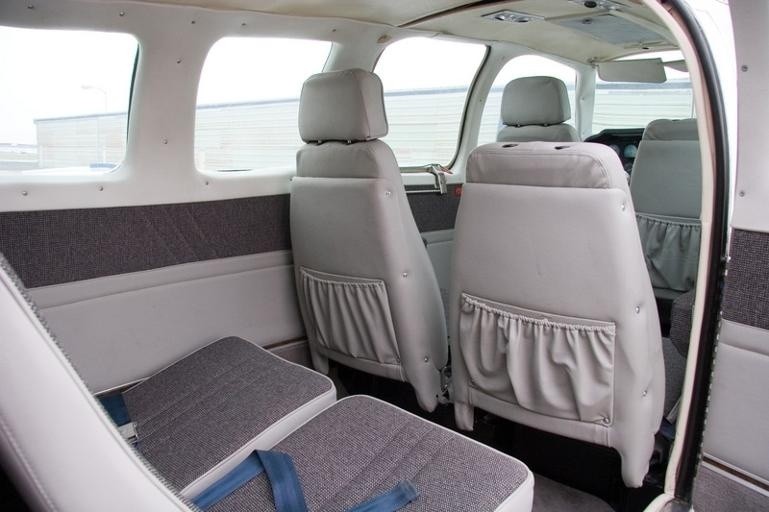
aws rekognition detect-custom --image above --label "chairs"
[454,136,663,484]
[1,248,536,512]
[639,121,706,307]
[477,78,586,135]
[284,79,450,398]
